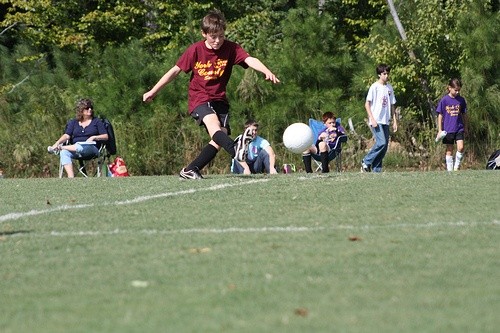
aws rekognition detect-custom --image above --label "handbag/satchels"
[107,157,128,177]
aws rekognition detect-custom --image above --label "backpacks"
[486,150,500,170]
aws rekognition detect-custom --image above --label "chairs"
[308,118,341,174]
[59,140,107,178]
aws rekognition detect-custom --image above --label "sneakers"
[360,158,371,173]
[231,126,257,161]
[47,145,60,155]
[179,167,204,179]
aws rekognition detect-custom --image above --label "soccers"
[283,123,314,154]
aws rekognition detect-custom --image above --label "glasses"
[84,106,93,110]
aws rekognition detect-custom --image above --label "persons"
[47,98,109,179]
[302,111,348,173]
[232,120,279,174]
[435,77,468,171]
[142,13,281,179]
[359,63,398,172]
